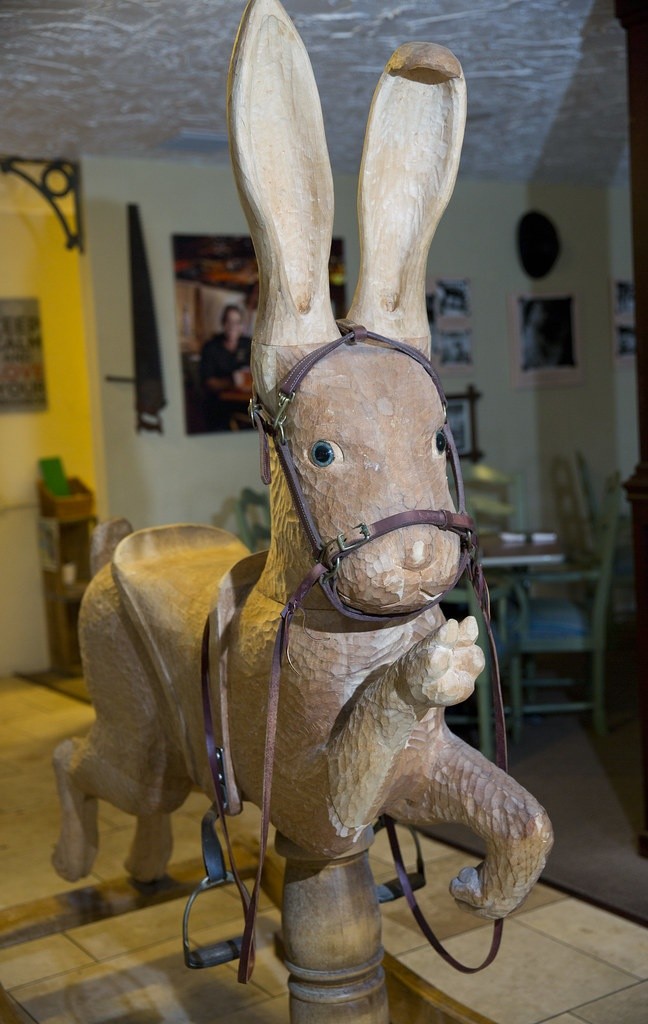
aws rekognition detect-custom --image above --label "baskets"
[36,477,94,520]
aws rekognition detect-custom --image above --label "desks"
[474,529,564,568]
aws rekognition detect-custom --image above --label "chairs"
[440,559,507,770]
[498,465,623,745]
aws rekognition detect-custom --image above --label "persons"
[519,301,561,371]
[198,303,253,391]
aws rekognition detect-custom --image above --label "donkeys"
[48,0,556,921]
